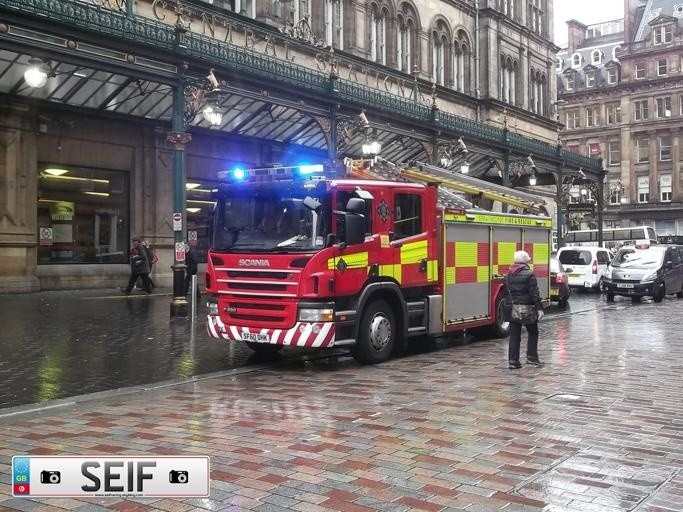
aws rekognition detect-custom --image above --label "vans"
[601,242,682,304]
[553,245,614,293]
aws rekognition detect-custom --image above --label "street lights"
[560,169,589,233]
[168,69,226,320]
[495,151,539,190]
[428,137,471,174]
[324,112,381,160]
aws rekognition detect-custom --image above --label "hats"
[131,236,147,244]
[512,250,533,264]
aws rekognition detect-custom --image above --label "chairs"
[258,199,293,238]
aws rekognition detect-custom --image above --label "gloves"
[501,321,510,330]
[538,309,544,320]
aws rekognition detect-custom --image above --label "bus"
[562,225,658,252]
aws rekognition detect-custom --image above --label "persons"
[121,238,152,297]
[185,242,200,298]
[136,241,155,291]
[501,251,545,369]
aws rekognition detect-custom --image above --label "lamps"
[580,180,588,196]
[203,105,215,123]
[460,159,469,174]
[362,134,370,155]
[529,169,537,186]
[210,101,223,125]
[440,154,453,168]
[370,136,381,154]
[23,58,48,88]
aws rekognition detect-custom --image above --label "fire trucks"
[202,147,556,365]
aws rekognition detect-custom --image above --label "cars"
[547,256,572,310]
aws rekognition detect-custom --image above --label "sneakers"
[120,283,154,295]
[524,359,546,368]
[507,360,522,370]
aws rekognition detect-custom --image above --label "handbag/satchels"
[131,254,159,266]
[511,304,537,326]
